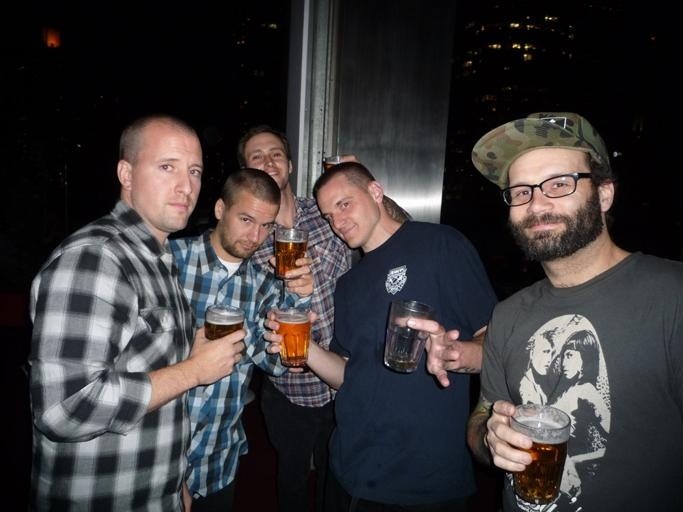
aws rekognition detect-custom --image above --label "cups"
[316,154,343,172]
[508,401,573,507]
[203,302,246,338]
[273,303,313,369]
[382,297,435,377]
[271,227,309,282]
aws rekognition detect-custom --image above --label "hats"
[472,112,612,190]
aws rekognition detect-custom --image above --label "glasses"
[500,172,592,207]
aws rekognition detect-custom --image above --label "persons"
[158,165,317,509]
[465,108,683,511]
[26,115,249,511]
[515,325,587,511]
[542,328,613,512]
[235,124,361,512]
[258,162,503,511]
